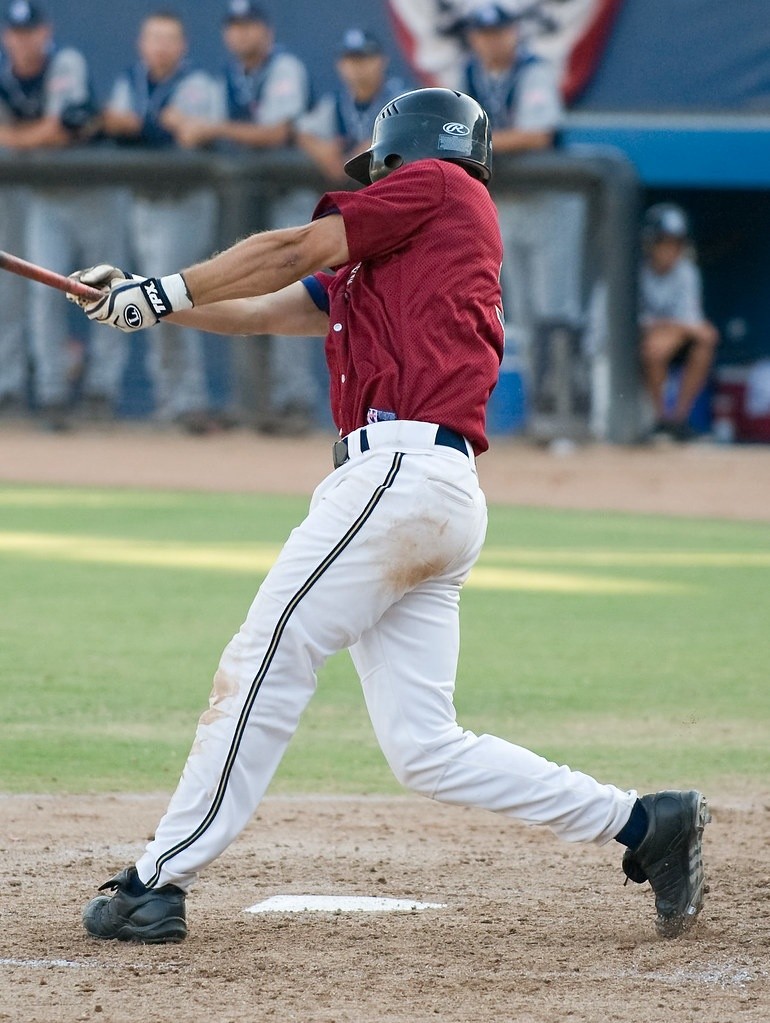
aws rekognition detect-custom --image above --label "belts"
[334,424,469,468]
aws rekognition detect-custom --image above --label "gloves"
[84,272,195,334]
[67,265,148,308]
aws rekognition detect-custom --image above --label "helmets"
[342,88,495,185]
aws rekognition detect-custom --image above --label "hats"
[5,0,41,26]
[641,201,689,236]
[337,29,382,55]
[470,0,519,24]
[222,0,261,22]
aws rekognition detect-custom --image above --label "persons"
[633,202,719,446]
[1,0,566,435]
[66,86,711,942]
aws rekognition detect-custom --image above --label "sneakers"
[622,789,712,938]
[80,867,188,943]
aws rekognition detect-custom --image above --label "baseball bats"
[0,250,113,302]
